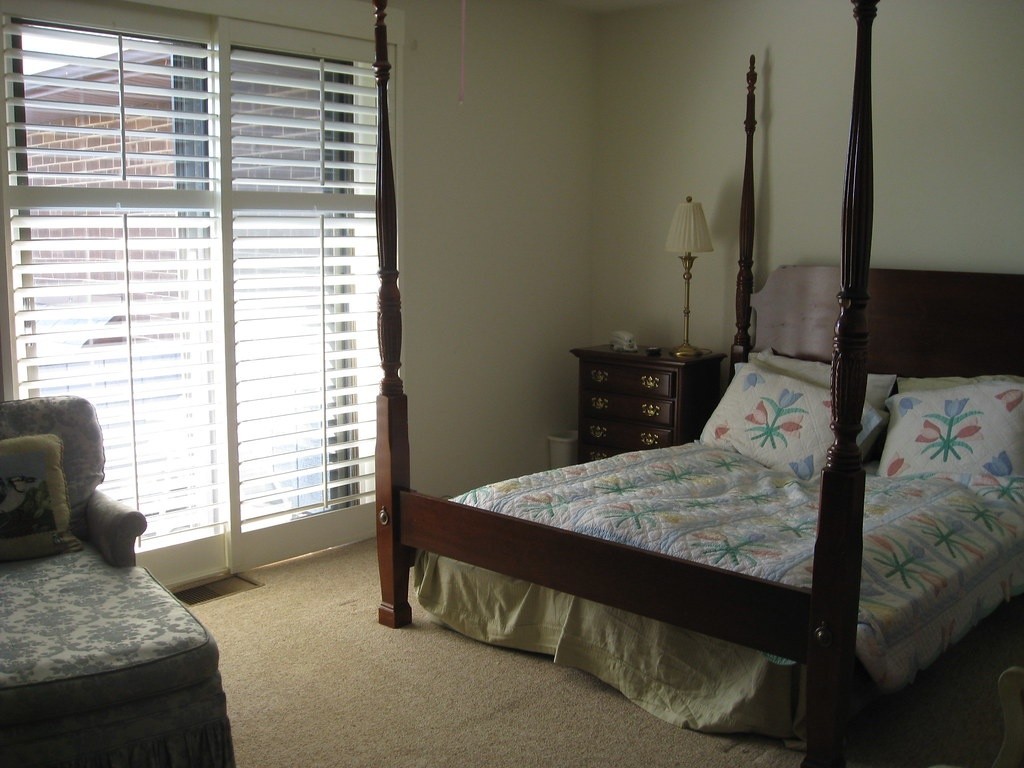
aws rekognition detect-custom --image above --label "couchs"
[0,397,236,768]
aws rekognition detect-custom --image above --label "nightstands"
[570,344,728,464]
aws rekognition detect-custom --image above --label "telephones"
[609,331,638,352]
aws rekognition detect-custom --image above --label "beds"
[372,0,1024,768]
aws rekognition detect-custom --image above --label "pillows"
[700,353,1024,479]
[0,433,83,563]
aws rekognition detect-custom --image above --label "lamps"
[664,197,714,356]
